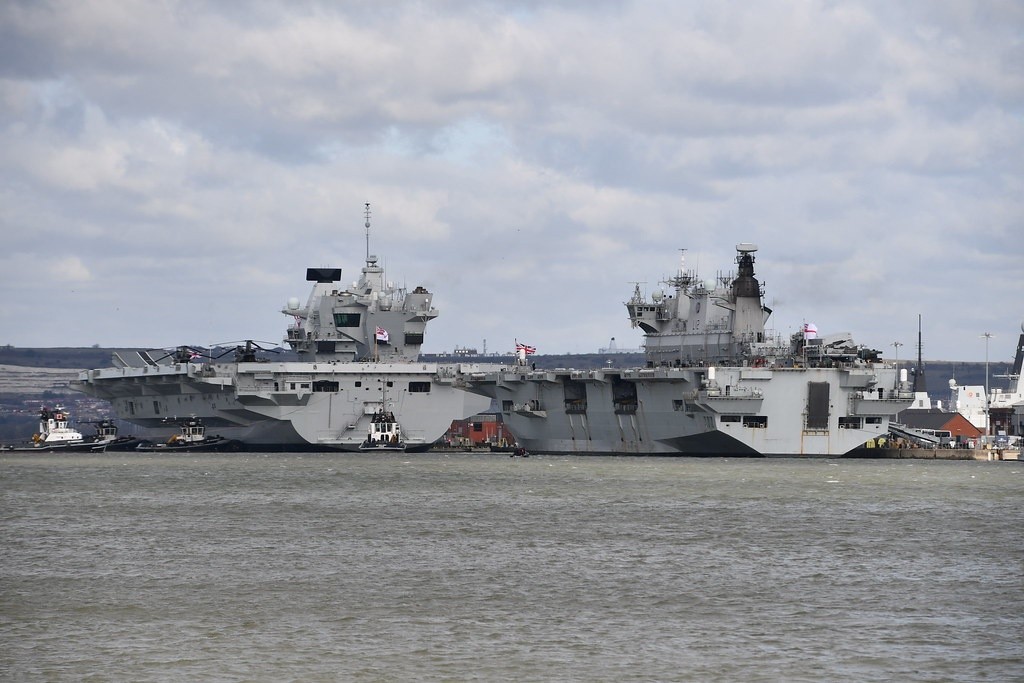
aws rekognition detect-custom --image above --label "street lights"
[978,332,994,436]
[890,341,903,423]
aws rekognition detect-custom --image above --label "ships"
[432,239,917,456]
[53,203,491,451]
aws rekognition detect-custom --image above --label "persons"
[532,362,535,370]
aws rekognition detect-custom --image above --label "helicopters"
[144,345,216,364]
[209,340,281,363]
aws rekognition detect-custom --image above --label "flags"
[516,343,536,354]
[804,324,816,339]
[376,327,388,340]
[190,349,202,359]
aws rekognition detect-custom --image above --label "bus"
[915,429,951,447]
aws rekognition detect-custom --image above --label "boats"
[358,377,407,452]
[489,438,519,452]
[88,418,137,451]
[133,418,230,451]
[0,401,110,453]
[432,429,490,452]
